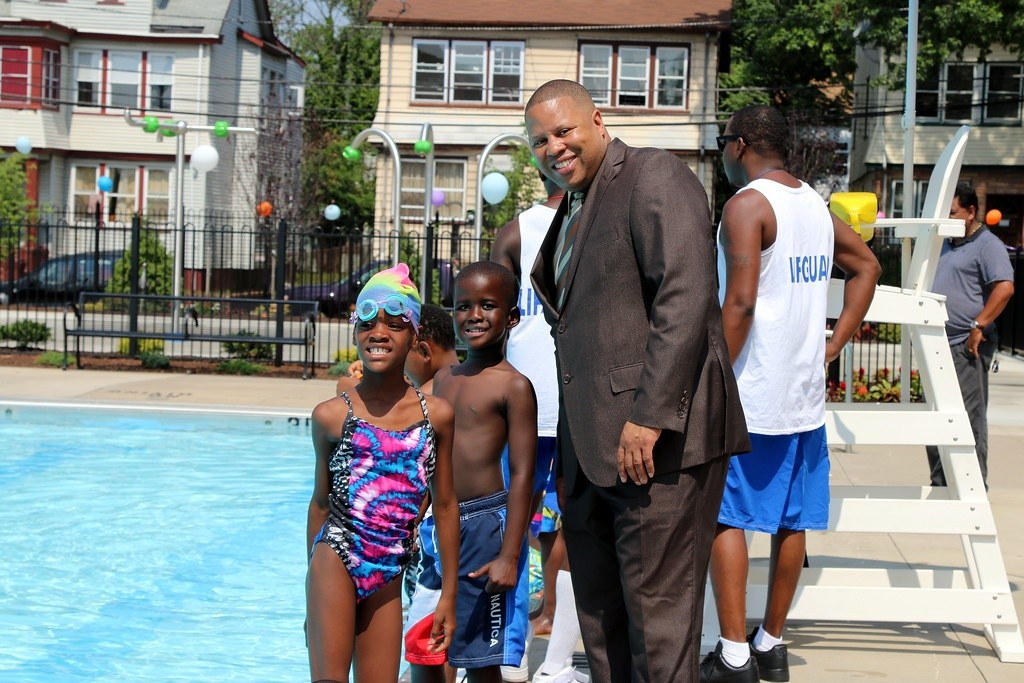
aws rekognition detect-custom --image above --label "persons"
[699,104,882,683]
[525,79,752,683]
[306,170,591,683]
[926,181,1014,492]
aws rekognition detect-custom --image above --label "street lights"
[92,173,111,292]
[474,131,540,263]
[254,196,340,366]
[343,128,402,271]
[414,122,444,302]
[123,102,258,333]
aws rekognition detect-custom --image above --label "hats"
[354,262,421,328]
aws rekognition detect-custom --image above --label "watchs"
[971,320,984,330]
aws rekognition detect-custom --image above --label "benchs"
[59,289,321,380]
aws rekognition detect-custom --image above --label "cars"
[216,255,462,321]
[0,248,129,311]
[0,249,162,308]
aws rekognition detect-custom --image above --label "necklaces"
[548,195,564,200]
[971,222,982,234]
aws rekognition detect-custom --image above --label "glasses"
[349,294,413,323]
[716,134,738,151]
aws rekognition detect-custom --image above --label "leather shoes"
[699,641,760,683]
[747,626,789,681]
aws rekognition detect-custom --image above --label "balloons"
[257,201,273,216]
[481,172,509,205]
[98,176,114,191]
[986,209,1002,225]
[324,204,341,220]
[432,190,445,207]
[191,144,220,172]
[16,137,32,154]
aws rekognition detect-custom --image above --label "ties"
[556,190,584,315]
